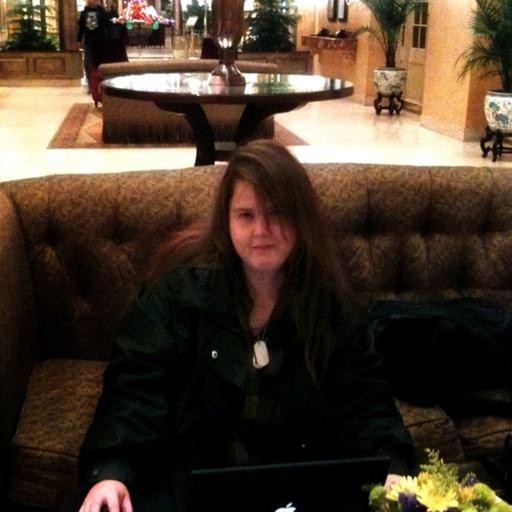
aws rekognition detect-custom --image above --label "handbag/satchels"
[367,298,512,419]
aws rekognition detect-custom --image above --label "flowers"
[362,447,512,512]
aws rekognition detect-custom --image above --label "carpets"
[47,103,310,149]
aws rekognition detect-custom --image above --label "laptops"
[186,455,392,512]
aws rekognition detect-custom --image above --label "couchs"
[98,59,280,144]
[0,163,512,512]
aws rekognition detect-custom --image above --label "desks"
[99,72,354,167]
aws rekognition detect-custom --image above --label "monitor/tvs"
[337,0,346,19]
[327,0,336,19]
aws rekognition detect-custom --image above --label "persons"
[104,20,128,63]
[77,1,109,95]
[107,5,119,20]
[74,138,420,512]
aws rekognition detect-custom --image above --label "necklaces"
[240,271,284,370]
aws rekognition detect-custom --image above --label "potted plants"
[454,0,512,133]
[350,0,424,96]
